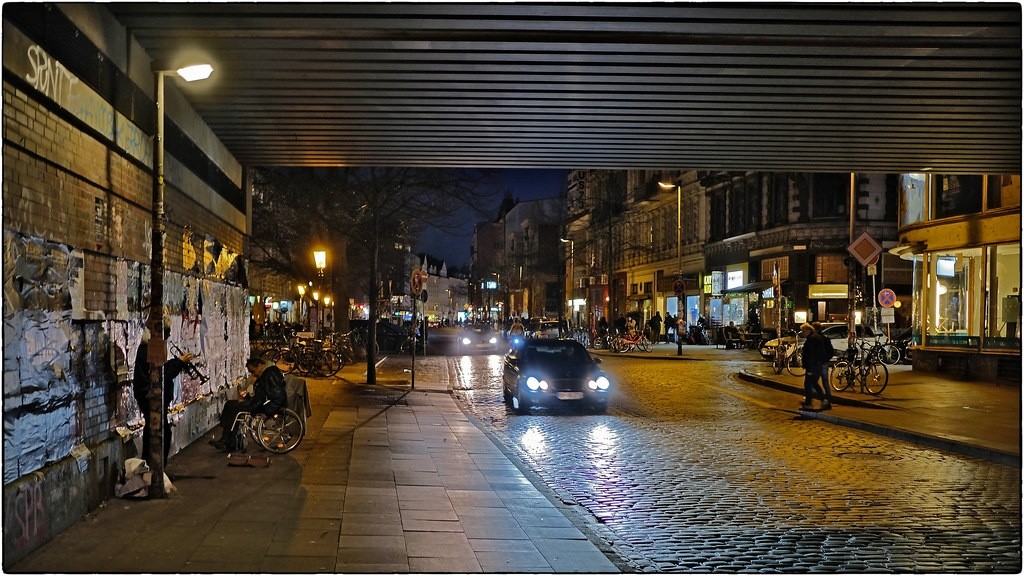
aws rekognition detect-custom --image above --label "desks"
[742,332,767,349]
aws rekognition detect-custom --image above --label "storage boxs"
[297,331,314,339]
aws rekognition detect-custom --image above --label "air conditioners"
[589,277,595,285]
[579,278,585,289]
[601,275,608,285]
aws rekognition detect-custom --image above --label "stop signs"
[420,271,428,283]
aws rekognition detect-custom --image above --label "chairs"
[723,327,741,349]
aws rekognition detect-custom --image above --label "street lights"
[147,64,214,499]
[658,182,682,355]
[446,289,450,311]
[561,239,573,318]
[313,251,327,339]
[492,272,500,285]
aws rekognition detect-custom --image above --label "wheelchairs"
[230,374,306,454]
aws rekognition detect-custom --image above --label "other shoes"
[208,440,243,451]
[819,404,832,410]
[801,402,811,406]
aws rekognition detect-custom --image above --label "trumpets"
[168,340,210,386]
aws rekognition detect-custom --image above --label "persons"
[597,317,608,349]
[509,318,525,337]
[653,311,663,344]
[800,322,835,410]
[625,316,636,337]
[133,315,193,483]
[724,321,748,348]
[664,312,673,344]
[697,312,706,330]
[208,357,288,452]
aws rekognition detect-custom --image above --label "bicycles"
[562,328,654,353]
[250,321,379,378]
[772,328,806,377]
[830,330,901,396]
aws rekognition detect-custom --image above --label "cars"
[350,321,420,354]
[456,324,499,356]
[892,328,912,366]
[503,337,610,416]
[761,323,891,365]
[524,317,565,339]
